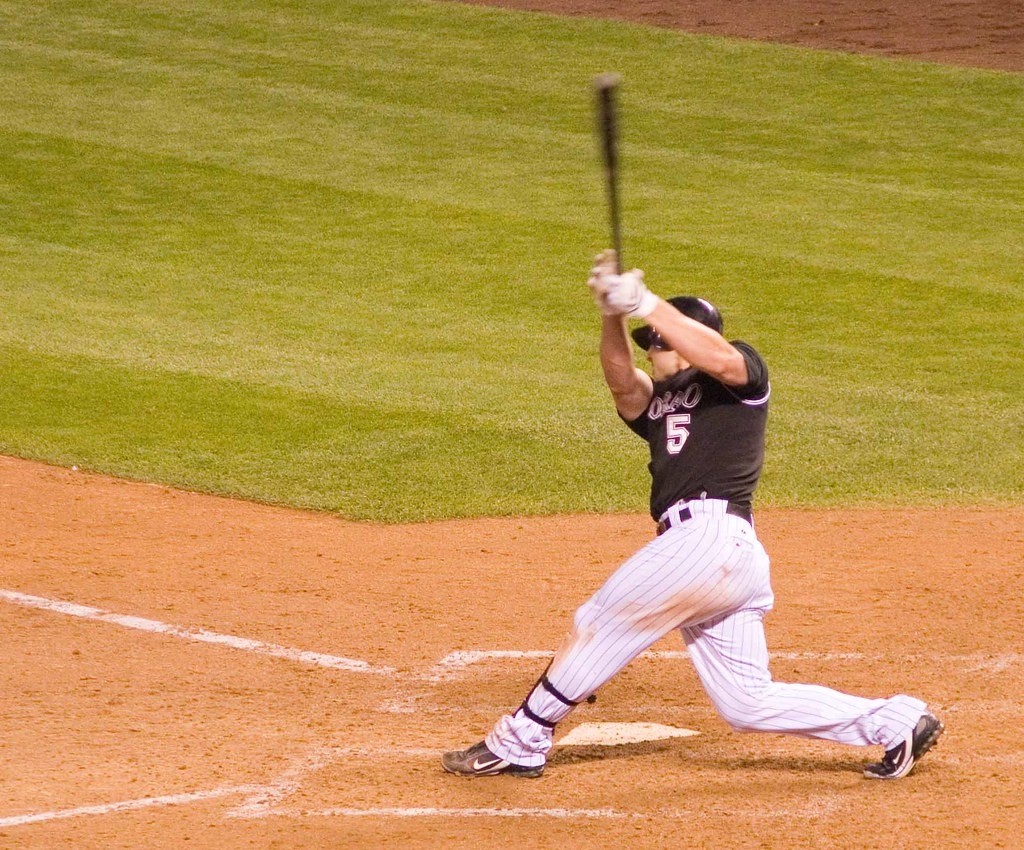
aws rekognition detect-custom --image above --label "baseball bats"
[591,72,625,274]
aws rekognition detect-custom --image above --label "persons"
[442,250,947,777]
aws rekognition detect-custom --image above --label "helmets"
[631,295,724,352]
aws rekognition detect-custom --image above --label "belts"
[655,504,752,537]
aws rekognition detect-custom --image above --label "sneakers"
[440,740,545,778]
[862,709,946,779]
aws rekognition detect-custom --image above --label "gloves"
[588,250,619,315]
[593,269,659,320]
